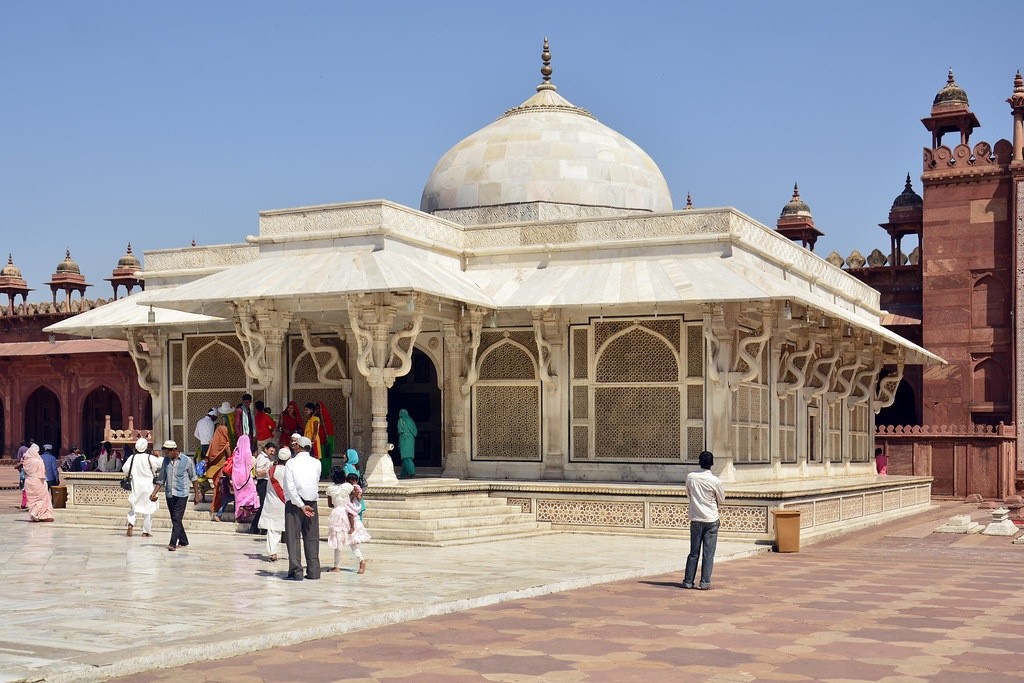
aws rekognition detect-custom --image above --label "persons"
[682,451,725,589]
[22,443,55,522]
[874,447,888,474]
[122,391,370,582]
[397,409,417,478]
[15,436,122,510]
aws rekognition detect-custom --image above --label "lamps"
[148,304,155,322]
[407,292,414,311]
[49,331,55,344]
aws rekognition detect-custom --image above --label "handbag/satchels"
[119,454,136,490]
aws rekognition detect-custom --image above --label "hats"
[218,402,235,414]
[163,440,177,448]
[135,438,148,452]
[278,448,291,461]
[292,437,312,452]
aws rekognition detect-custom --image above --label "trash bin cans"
[772,510,800,553]
[52,486,67,508]
